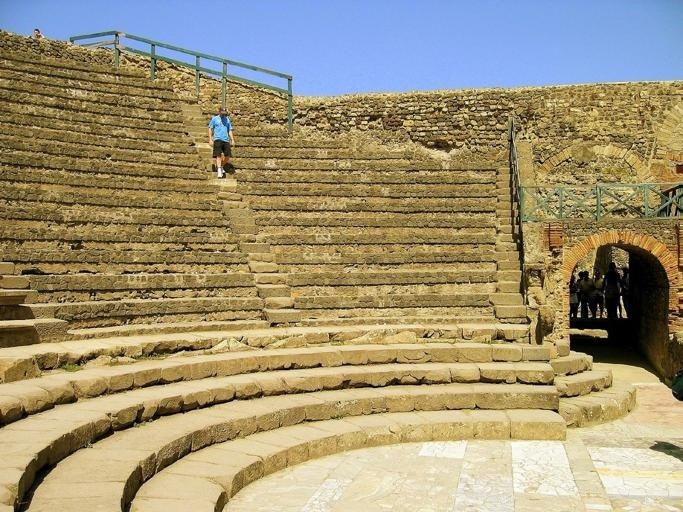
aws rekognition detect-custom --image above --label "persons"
[208,108,236,179]
[33,29,43,39]
[570,262,630,322]
